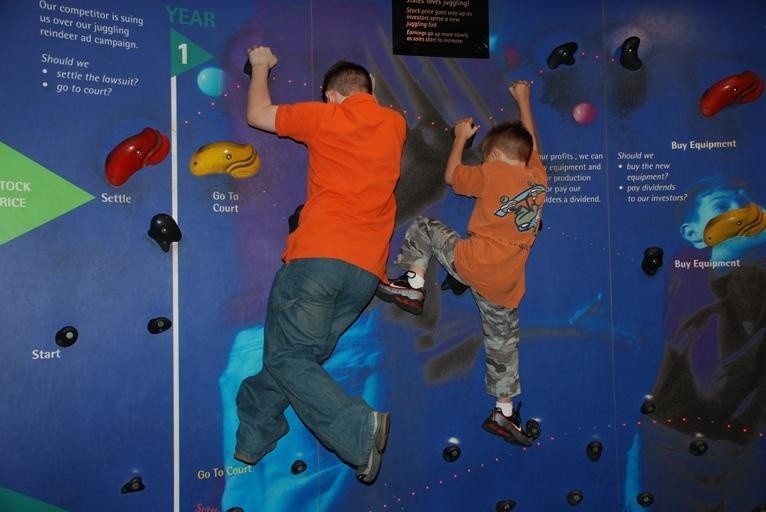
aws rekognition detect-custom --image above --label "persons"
[235,44,408,484]
[664,169,766,381]
[378,80,549,448]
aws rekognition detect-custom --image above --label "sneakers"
[482,402,533,448]
[376,271,427,316]
[357,411,391,484]
[234,444,277,466]
